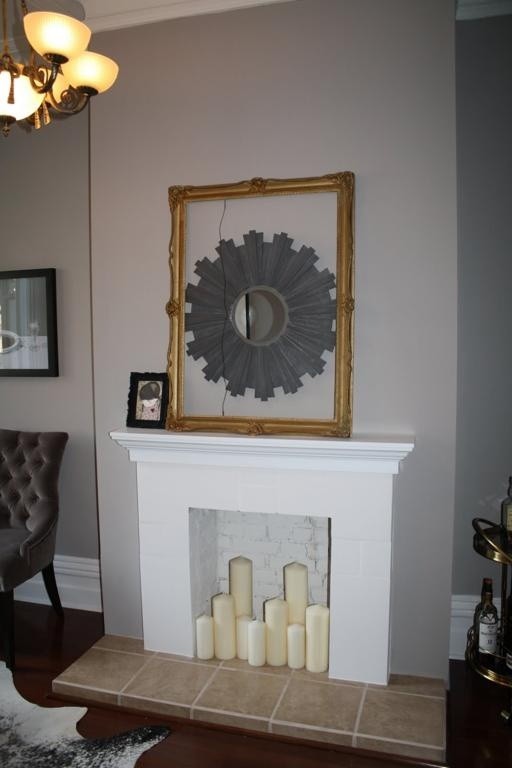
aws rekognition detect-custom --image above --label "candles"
[287,623,305,669]
[305,604,329,672]
[235,616,252,660]
[196,615,214,659]
[284,561,308,625]
[264,598,288,667]
[213,593,236,660]
[248,620,266,667]
[229,556,253,619]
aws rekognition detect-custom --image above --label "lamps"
[0,1,120,139]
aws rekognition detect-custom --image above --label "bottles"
[473,577,498,657]
[500,474,512,551]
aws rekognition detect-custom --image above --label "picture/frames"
[164,171,357,440]
[125,371,170,430]
[0,268,60,379]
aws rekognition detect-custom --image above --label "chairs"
[1,428,68,678]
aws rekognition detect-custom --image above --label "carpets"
[0,662,170,768]
